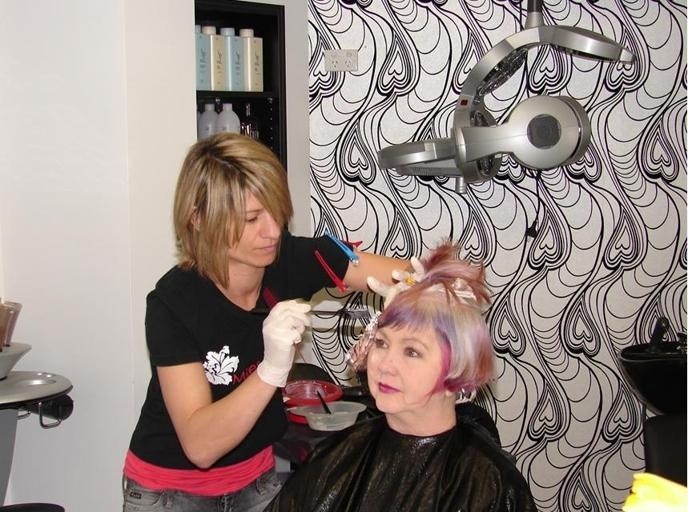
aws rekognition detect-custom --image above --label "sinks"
[613,338,687,415]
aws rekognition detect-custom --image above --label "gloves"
[365,255,427,313]
[257,299,312,389]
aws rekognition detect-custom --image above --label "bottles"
[239,100,263,139]
[197,102,218,139]
[217,102,239,138]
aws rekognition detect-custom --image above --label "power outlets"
[325,49,359,73]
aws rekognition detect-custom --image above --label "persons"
[121,132,428,512]
[261,240,541,512]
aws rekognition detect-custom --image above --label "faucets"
[645,316,670,352]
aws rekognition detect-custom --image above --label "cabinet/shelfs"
[195,0,287,193]
